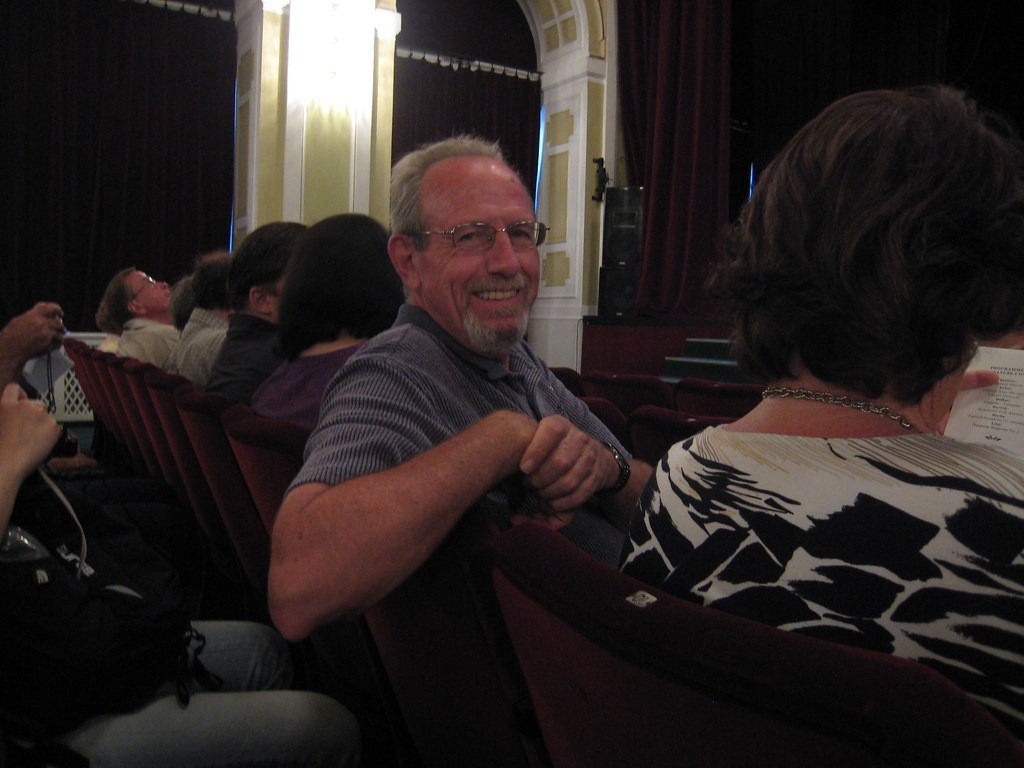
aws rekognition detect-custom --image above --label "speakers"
[598,186,649,325]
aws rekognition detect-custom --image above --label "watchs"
[599,441,631,496]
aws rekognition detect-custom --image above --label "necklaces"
[760,384,911,430]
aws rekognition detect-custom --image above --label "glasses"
[128,276,156,303]
[410,221,551,252]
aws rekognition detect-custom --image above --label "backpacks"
[0,472,222,735]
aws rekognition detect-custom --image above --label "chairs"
[64,338,1024,768]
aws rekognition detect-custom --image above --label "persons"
[91,249,238,386]
[616,80,1024,745]
[247,212,407,431]
[0,302,361,768]
[264,134,656,644]
[205,223,309,400]
